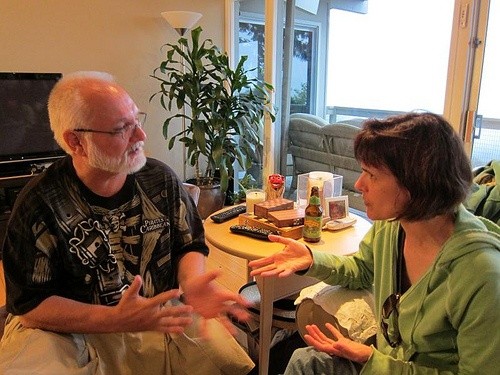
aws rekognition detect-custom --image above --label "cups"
[245,189,265,214]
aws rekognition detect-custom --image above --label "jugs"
[306,169,334,222]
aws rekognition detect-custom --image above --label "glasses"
[381,293,402,347]
[73,112,146,141]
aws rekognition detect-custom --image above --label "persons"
[248,107,500,375]
[0,70,256,374]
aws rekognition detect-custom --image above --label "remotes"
[210,205,246,223]
[231,225,275,240]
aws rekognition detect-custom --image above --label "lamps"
[161,10,203,182]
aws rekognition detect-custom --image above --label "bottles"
[303,186,322,243]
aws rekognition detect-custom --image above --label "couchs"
[294,160,500,348]
[289,112,370,213]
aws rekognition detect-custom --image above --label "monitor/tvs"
[0,71,65,173]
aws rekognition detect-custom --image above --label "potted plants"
[149,27,280,220]
[222,173,258,203]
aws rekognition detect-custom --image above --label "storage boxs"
[297,172,344,217]
[255,198,294,218]
[239,212,330,240]
[268,209,305,227]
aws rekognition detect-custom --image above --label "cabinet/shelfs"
[0,170,42,260]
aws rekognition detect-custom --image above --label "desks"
[203,202,373,375]
